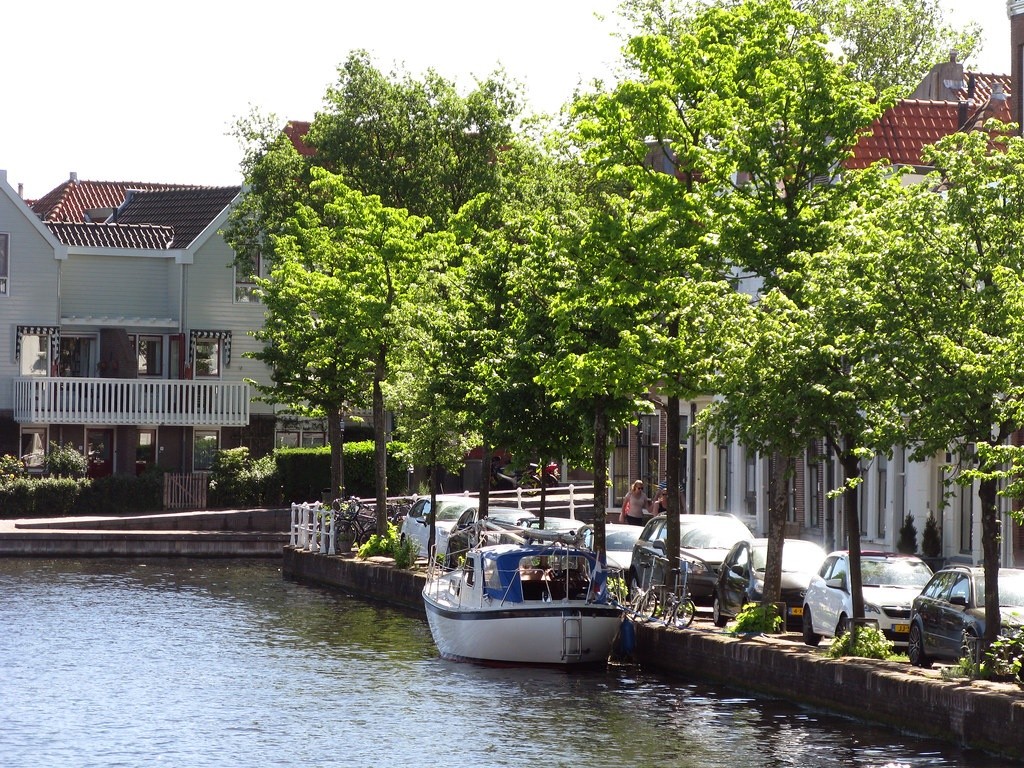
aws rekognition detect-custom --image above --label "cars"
[444,506,536,574]
[907,564,1024,669]
[711,538,829,632]
[504,514,593,568]
[569,522,653,586]
[399,494,481,569]
[801,549,936,656]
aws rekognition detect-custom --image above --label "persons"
[619,480,648,527]
[648,471,686,516]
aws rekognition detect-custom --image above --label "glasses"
[636,486,644,490]
[662,494,668,496]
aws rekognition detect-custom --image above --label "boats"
[420,515,623,669]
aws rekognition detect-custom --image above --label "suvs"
[628,511,755,617]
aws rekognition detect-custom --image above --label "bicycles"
[330,492,420,556]
[628,551,669,624]
[655,555,704,631]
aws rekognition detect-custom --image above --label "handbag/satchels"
[625,493,632,517]
